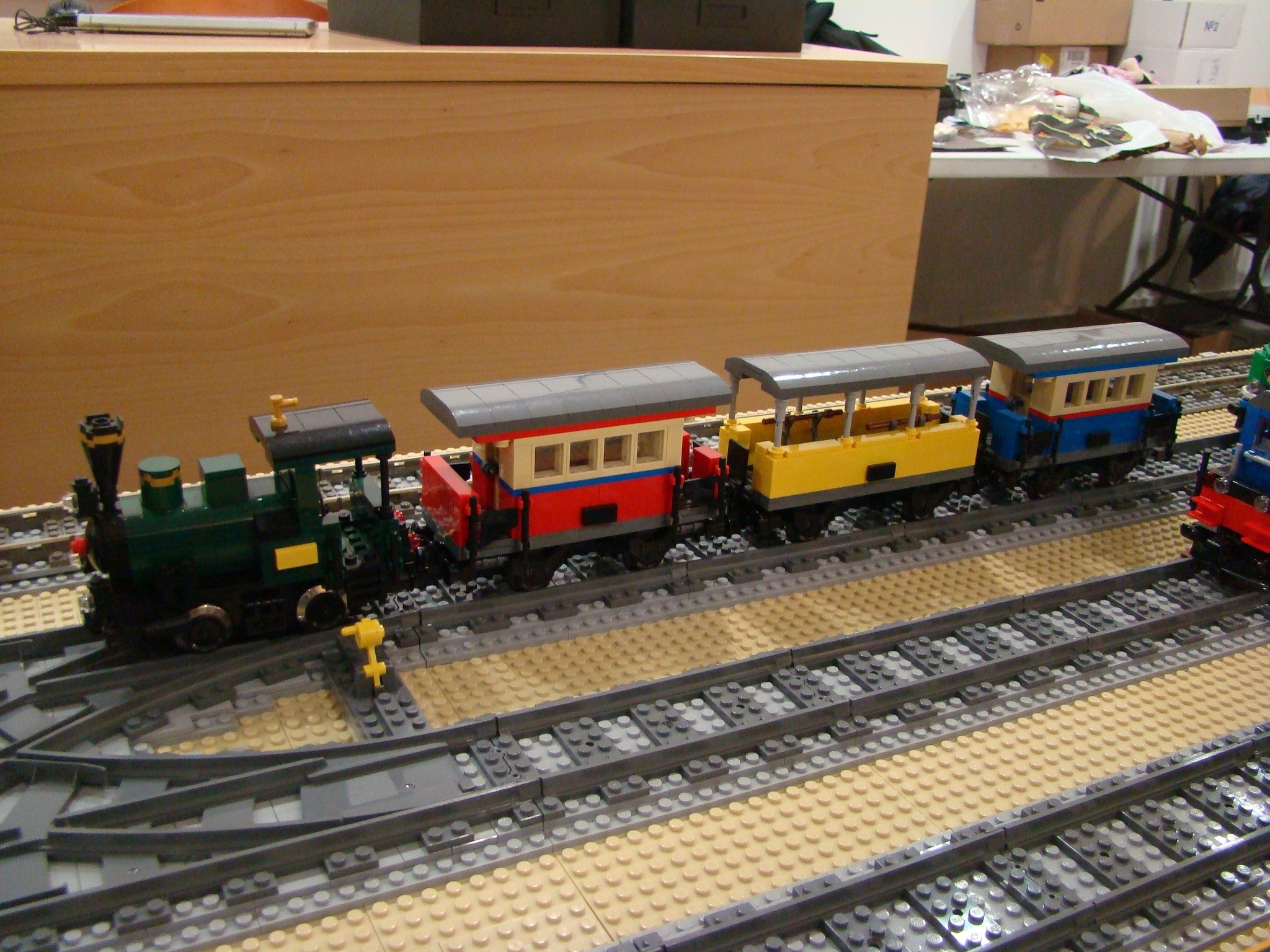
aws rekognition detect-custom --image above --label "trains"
[63,321,1192,660]
[1181,345,1270,593]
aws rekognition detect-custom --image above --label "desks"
[0,20,945,508]
[919,128,1270,329]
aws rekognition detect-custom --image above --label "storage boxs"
[1127,0,1188,48]
[1181,0,1246,49]
[1116,49,1232,84]
[974,0,1131,46]
[985,46,1108,75]
